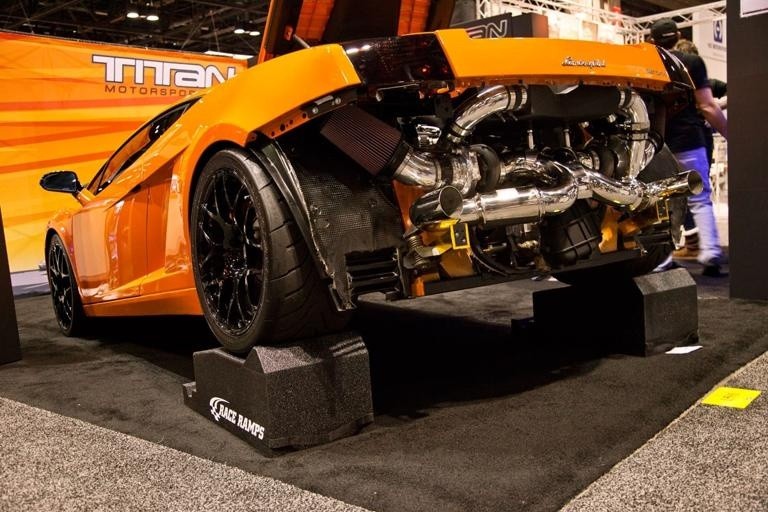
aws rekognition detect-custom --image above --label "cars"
[36,0,711,360]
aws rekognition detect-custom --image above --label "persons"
[646,18,728,276]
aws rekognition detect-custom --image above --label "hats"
[652,18,679,38]
